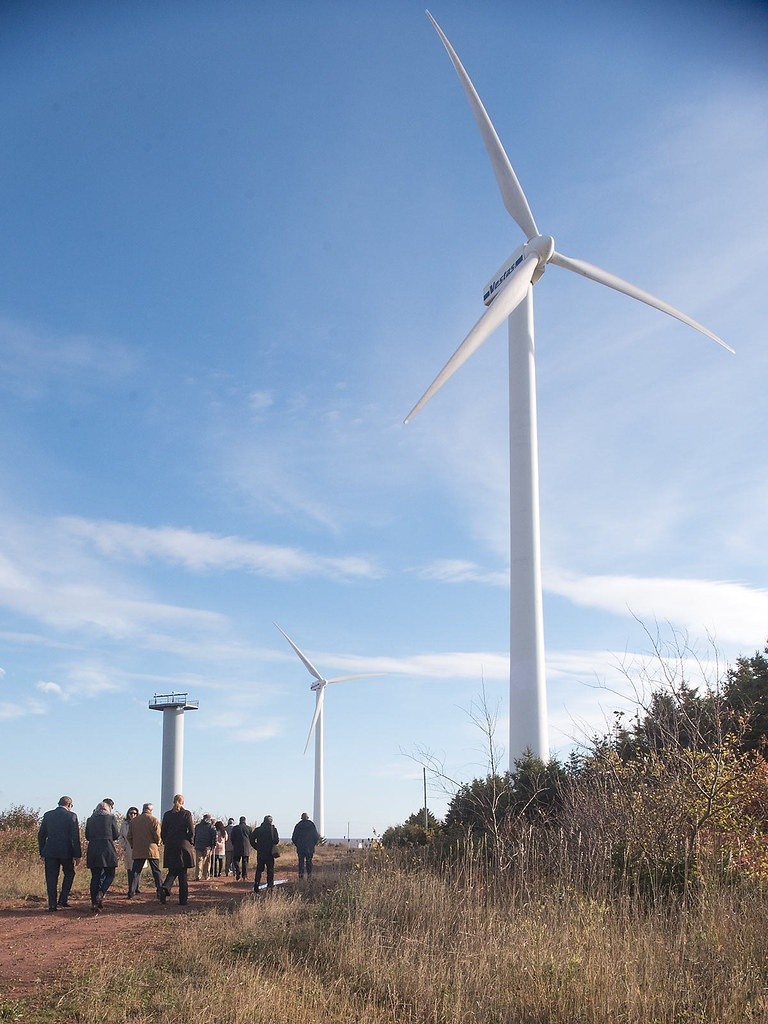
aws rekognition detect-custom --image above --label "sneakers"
[49,908,55,912]
[55,902,73,910]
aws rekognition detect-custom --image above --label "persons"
[85,802,119,912]
[292,812,319,882]
[127,803,164,899]
[38,796,82,911]
[191,813,254,882]
[103,798,114,812]
[120,806,142,895]
[159,794,194,907]
[249,815,279,894]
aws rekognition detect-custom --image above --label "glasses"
[112,806,114,809]
[129,811,136,815]
[68,803,74,808]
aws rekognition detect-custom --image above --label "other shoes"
[253,881,260,893]
[236,875,241,880]
[243,877,248,880]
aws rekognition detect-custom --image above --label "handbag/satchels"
[272,844,281,858]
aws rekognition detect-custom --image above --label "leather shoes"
[214,873,221,877]
[159,889,166,904]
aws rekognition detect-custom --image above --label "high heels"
[92,891,103,913]
